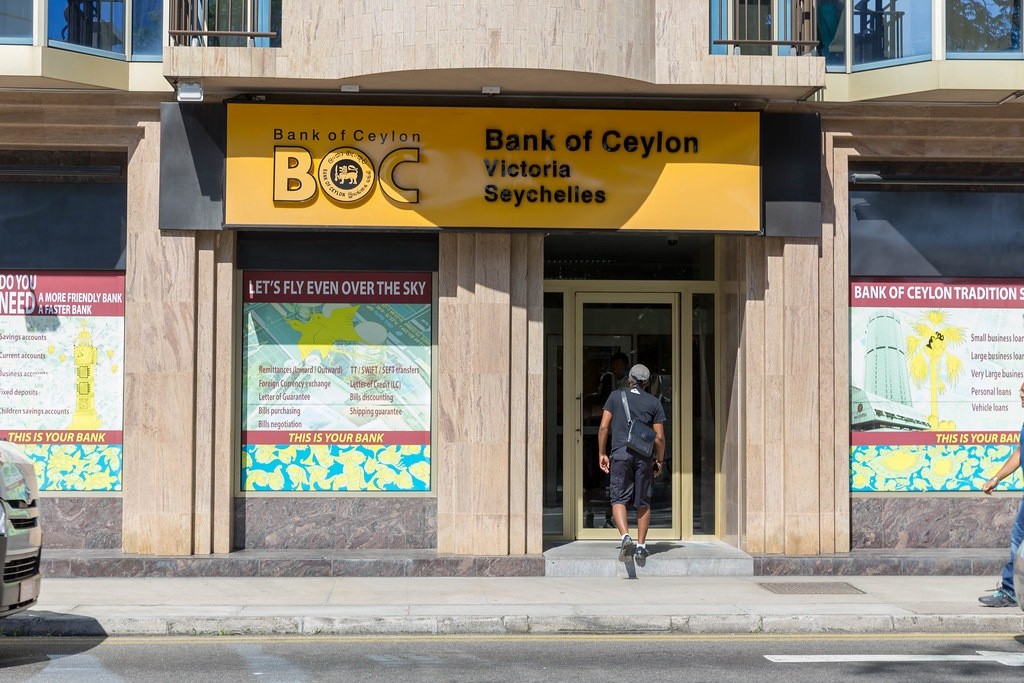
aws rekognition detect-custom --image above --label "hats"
[629,364,650,385]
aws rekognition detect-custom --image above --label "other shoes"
[618,535,633,562]
[635,544,650,560]
[604,520,615,528]
[585,513,595,528]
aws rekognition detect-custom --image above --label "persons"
[583,351,631,528]
[977,383,1024,607]
[598,363,667,561]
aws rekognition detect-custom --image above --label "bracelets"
[658,461,663,463]
[992,477,1000,483]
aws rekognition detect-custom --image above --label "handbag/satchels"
[625,419,657,457]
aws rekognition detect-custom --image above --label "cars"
[0,438,45,624]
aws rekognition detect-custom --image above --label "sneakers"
[978,580,1019,607]
